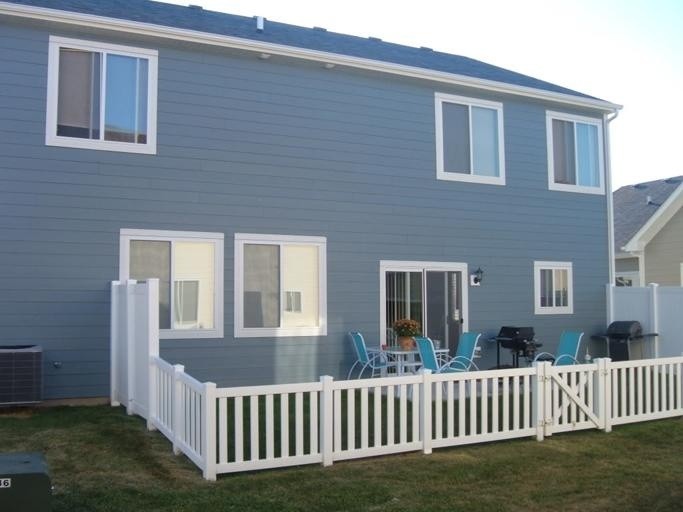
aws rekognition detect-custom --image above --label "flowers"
[393,320,421,337]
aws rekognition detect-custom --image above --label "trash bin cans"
[606,321,643,361]
[1,454,52,511]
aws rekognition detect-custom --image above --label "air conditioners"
[0,345,42,408]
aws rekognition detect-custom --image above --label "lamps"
[474,266,484,283]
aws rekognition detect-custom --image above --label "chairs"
[347,327,584,399]
